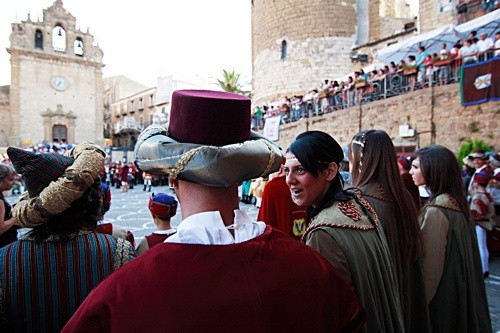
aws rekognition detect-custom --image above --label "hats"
[468,148,484,158]
[148,191,178,216]
[462,155,475,167]
[490,154,500,166]
[134,89,286,186]
[101,182,111,210]
[6,140,106,228]
[475,171,489,183]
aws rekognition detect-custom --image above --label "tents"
[454,9,500,47]
[373,24,467,65]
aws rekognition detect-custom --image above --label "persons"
[254,30,500,129]
[258,157,307,242]
[0,142,139,333]
[0,165,17,247]
[283,131,404,333]
[348,130,421,333]
[96,183,134,249]
[409,146,494,333]
[470,166,495,278]
[59,89,366,333]
[237,149,500,260]
[0,143,154,195]
[135,192,177,255]
